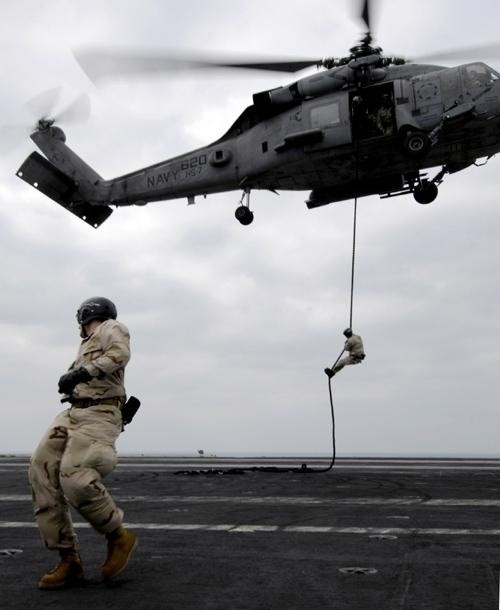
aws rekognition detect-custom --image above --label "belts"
[61,397,125,409]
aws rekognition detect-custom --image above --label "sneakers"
[324,367,335,378]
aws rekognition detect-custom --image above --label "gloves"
[57,366,93,396]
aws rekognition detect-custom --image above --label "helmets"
[76,296,117,324]
[343,328,352,335]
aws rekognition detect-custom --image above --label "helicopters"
[11,0,500,231]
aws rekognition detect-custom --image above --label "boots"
[100,525,138,578]
[37,545,82,589]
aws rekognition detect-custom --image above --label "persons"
[350,94,377,126]
[26,294,145,590]
[377,89,395,136]
[324,327,366,380]
